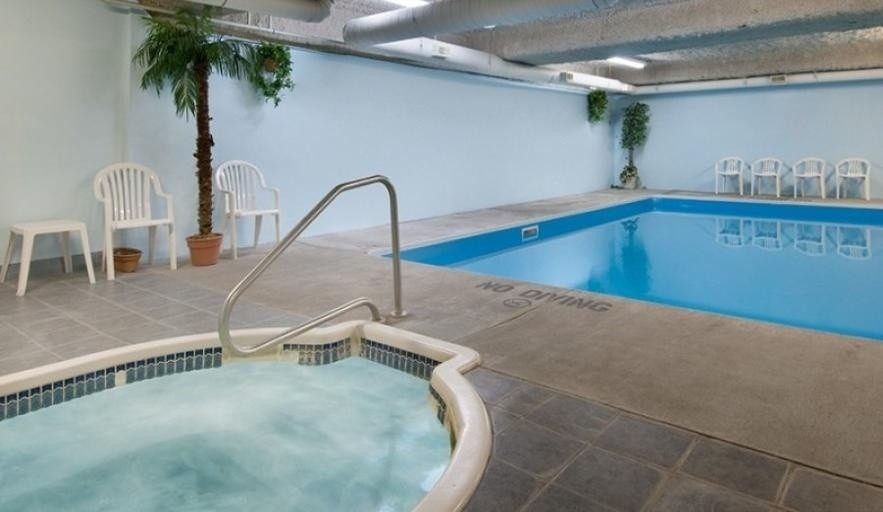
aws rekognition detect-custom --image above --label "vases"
[111,247,142,273]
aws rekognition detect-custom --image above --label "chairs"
[712,156,871,202]
[93,162,176,283]
[215,158,283,262]
[712,216,872,261]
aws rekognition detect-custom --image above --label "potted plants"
[618,99,651,189]
[136,0,294,267]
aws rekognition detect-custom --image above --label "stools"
[0,221,97,297]
